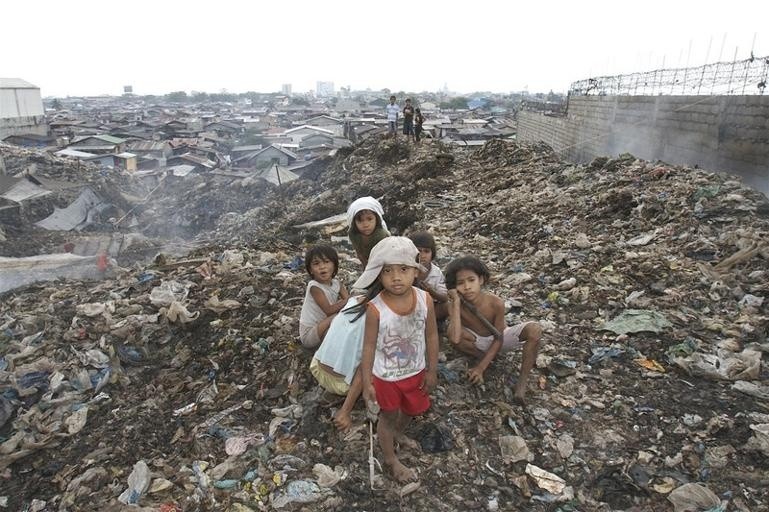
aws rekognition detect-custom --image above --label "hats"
[350,236,429,289]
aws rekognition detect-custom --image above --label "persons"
[351,235,440,485]
[299,244,350,349]
[444,255,542,406]
[386,95,400,136]
[407,231,449,321]
[414,108,426,141]
[402,98,414,134]
[346,196,392,269]
[308,296,369,397]
